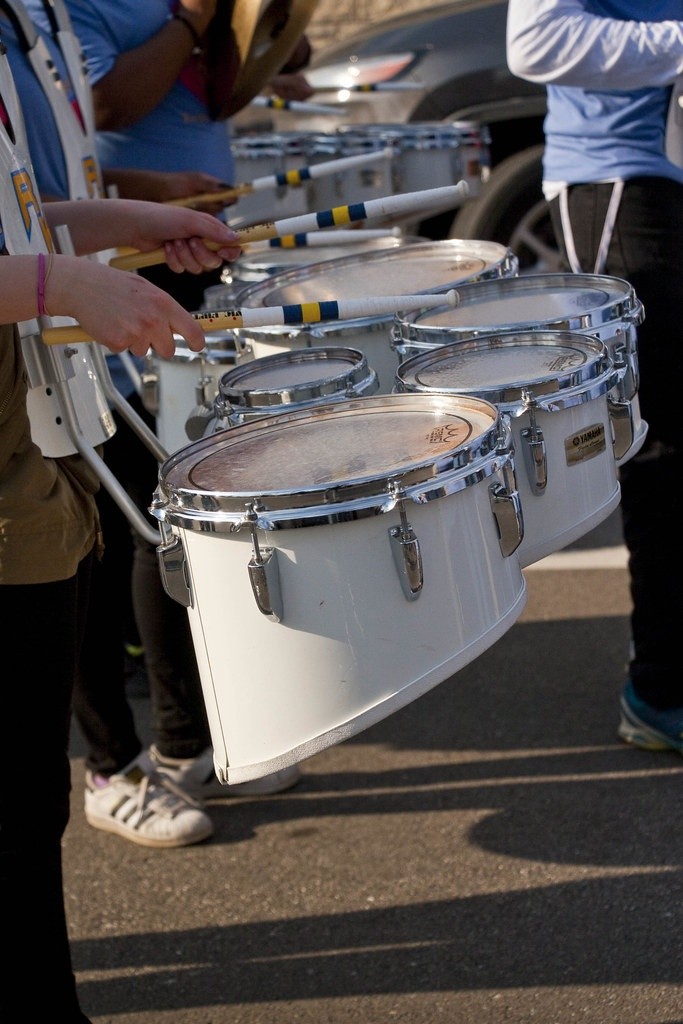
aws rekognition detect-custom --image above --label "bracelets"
[166,12,203,54]
[38,253,45,317]
[44,253,54,317]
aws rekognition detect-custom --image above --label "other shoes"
[617,680,683,751]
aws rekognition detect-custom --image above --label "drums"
[146,116,653,790]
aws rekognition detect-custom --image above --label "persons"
[0,0,316,1024]
[506,0,683,750]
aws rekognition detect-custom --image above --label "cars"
[298,0,572,275]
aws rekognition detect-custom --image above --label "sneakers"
[148,744,300,794]
[85,751,213,847]
